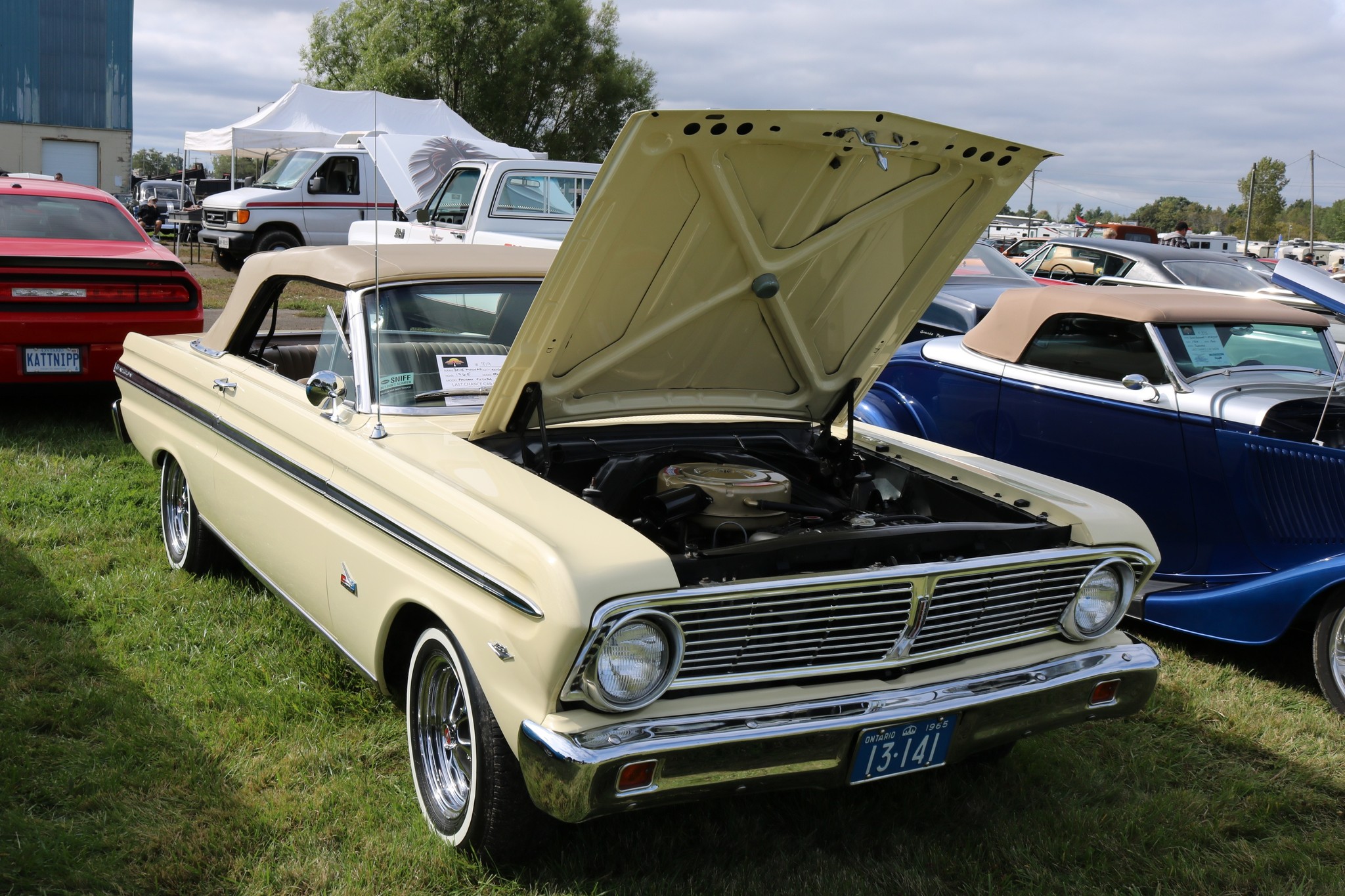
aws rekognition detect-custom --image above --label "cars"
[898,239,1054,350]
[1016,238,1345,382]
[0,174,206,385]
[963,218,1345,296]
[114,110,1164,854]
[133,179,198,230]
[843,278,1345,712]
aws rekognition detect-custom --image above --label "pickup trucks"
[348,156,610,262]
[198,129,464,266]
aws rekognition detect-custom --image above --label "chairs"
[327,171,347,194]
[133,207,162,241]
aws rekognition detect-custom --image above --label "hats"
[148,196,158,203]
[1176,222,1192,230]
[1305,253,1315,256]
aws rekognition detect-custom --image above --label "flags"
[1274,234,1283,258]
[1075,214,1087,227]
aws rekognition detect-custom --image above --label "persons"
[1088,228,1125,277]
[137,196,162,241]
[1010,238,1019,253]
[55,173,65,182]
[1330,261,1343,282]
[1159,222,1190,249]
[1300,253,1316,266]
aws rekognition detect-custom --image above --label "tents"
[176,84,498,257]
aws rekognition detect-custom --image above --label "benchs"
[263,343,510,409]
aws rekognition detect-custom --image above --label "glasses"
[151,199,157,202]
[55,176,62,179]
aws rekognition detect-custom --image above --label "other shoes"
[150,235,159,241]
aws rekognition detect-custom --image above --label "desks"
[168,219,215,263]
